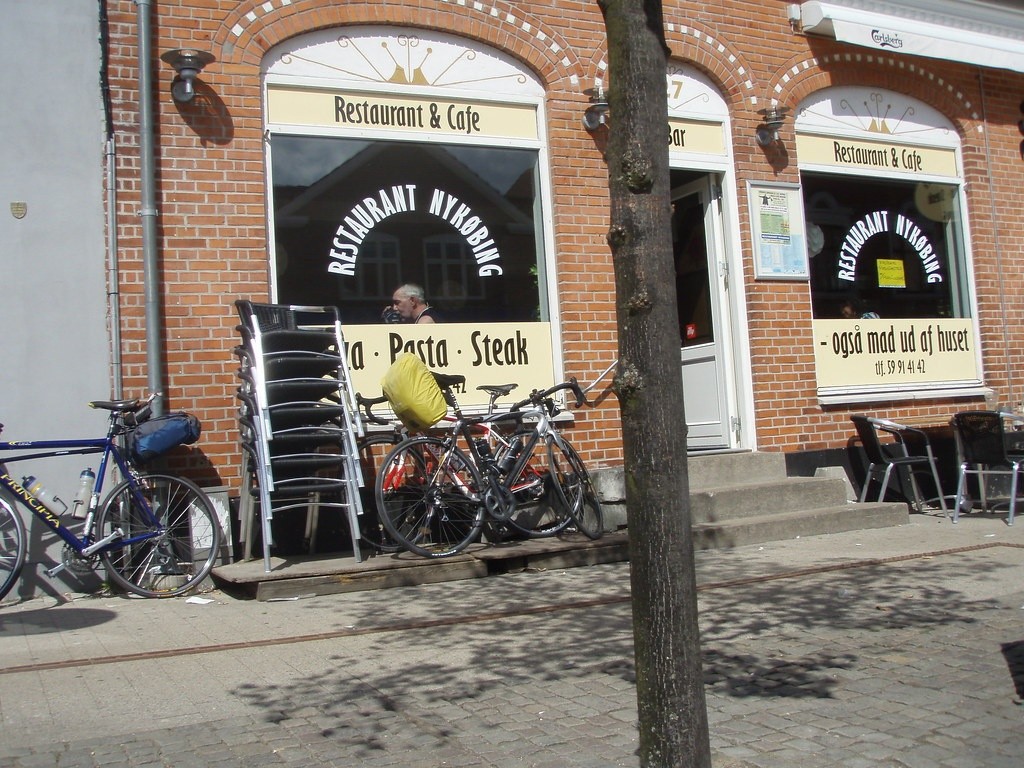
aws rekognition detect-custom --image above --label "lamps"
[757,107,791,146]
[162,49,216,102]
[582,87,609,129]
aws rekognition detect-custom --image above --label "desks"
[893,426,1024,512]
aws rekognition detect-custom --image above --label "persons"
[379,283,450,324]
[837,293,880,319]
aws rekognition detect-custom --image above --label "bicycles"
[338,371,605,560]
[0,398,222,603]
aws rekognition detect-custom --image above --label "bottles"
[72,467,95,519]
[21,476,68,516]
[497,435,523,475]
[436,444,465,472]
[1017,401,1023,413]
[475,437,498,476]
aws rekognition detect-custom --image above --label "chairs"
[229,300,367,573]
[948,410,1024,526]
[850,412,951,520]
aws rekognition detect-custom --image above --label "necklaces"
[415,307,432,323]
